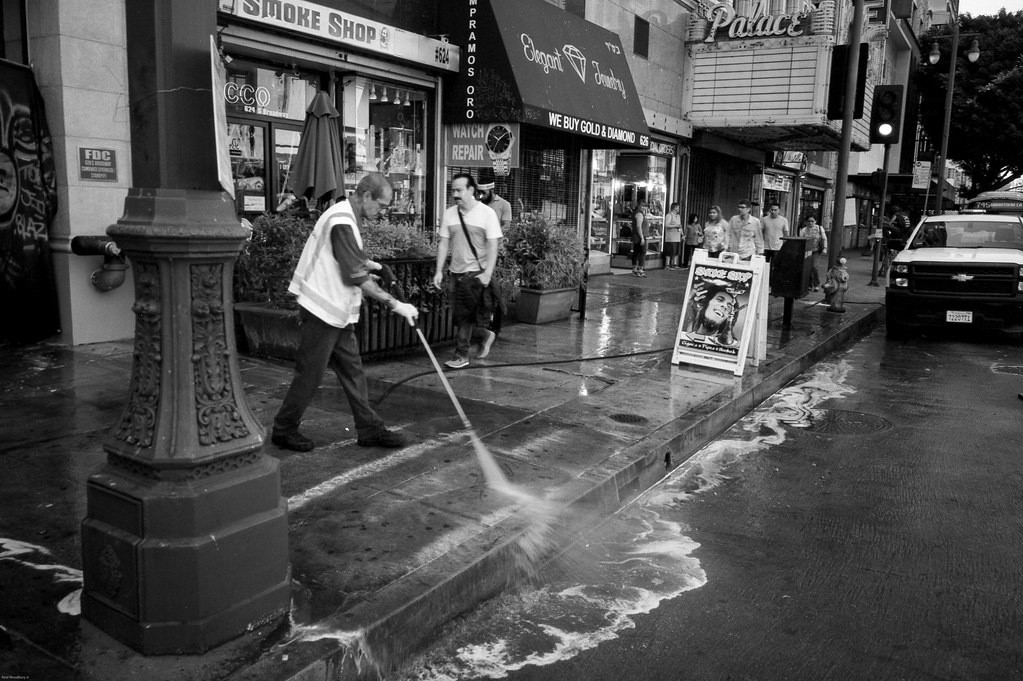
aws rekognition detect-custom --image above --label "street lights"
[929,26,981,216]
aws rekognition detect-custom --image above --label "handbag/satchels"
[482,275,498,309]
[698,233,704,243]
[819,225,824,253]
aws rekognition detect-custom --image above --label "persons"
[728,200,764,261]
[632,201,650,277]
[270,174,419,452]
[702,205,729,258]
[887,206,909,239]
[665,202,684,269]
[761,202,789,263]
[683,214,703,268]
[694,284,739,336]
[799,214,828,292]
[434,173,512,369]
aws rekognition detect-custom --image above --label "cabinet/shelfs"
[590,178,668,270]
[344,169,424,215]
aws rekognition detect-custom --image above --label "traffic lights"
[869,84,903,144]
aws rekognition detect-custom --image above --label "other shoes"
[664,266,675,271]
[814,286,819,292]
[632,270,647,278]
[808,286,811,290]
[672,264,680,269]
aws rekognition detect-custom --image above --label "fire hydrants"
[821,257,850,312]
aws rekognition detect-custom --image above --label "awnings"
[390,0,650,150]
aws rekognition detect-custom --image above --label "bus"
[964,191,1023,214]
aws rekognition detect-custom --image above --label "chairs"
[989,228,1021,248]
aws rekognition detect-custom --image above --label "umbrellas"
[286,91,348,220]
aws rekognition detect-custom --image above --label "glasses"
[736,206,748,209]
[477,189,486,195]
[362,190,390,210]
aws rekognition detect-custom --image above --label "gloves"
[391,300,419,327]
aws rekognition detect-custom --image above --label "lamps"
[369,84,377,99]
[403,92,410,105]
[393,90,400,104]
[380,87,388,101]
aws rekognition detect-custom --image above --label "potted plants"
[502,207,592,325]
[232,212,524,366]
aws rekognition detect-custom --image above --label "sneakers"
[444,353,469,369]
[271,431,313,452]
[358,429,405,447]
[475,330,495,358]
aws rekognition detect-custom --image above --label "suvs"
[886,214,1023,341]
